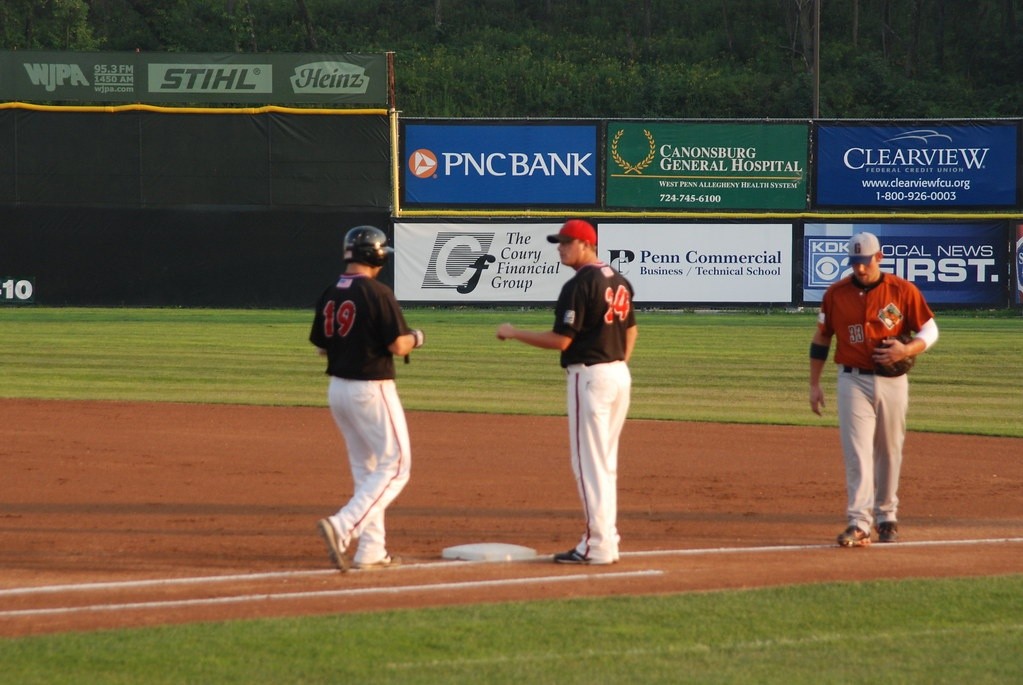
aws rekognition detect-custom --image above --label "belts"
[844,367,880,375]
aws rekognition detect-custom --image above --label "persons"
[307,225,425,572]
[497,219,637,565]
[808,231,941,547]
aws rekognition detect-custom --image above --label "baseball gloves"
[876,336,915,375]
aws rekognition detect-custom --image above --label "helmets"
[342,225,394,266]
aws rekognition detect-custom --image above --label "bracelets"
[410,332,418,349]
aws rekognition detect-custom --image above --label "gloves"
[408,329,426,348]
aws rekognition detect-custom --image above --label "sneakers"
[353,553,401,568]
[318,517,351,577]
[554,548,593,564]
[838,525,871,546]
[879,521,897,541]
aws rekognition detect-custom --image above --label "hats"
[547,220,597,246]
[847,232,880,265]
